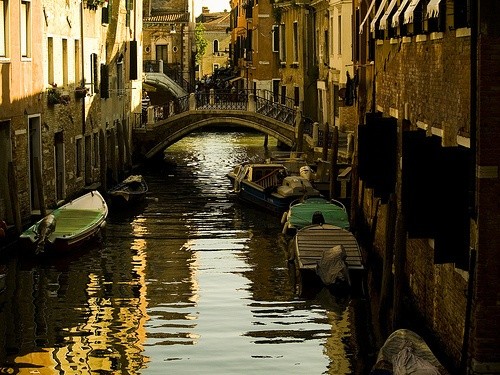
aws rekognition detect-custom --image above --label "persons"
[142,70,314,136]
[34,214,57,255]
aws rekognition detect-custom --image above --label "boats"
[19,190,108,251]
[227,163,324,222]
[280,197,365,300]
[110,174,150,201]
[371,328,450,375]
